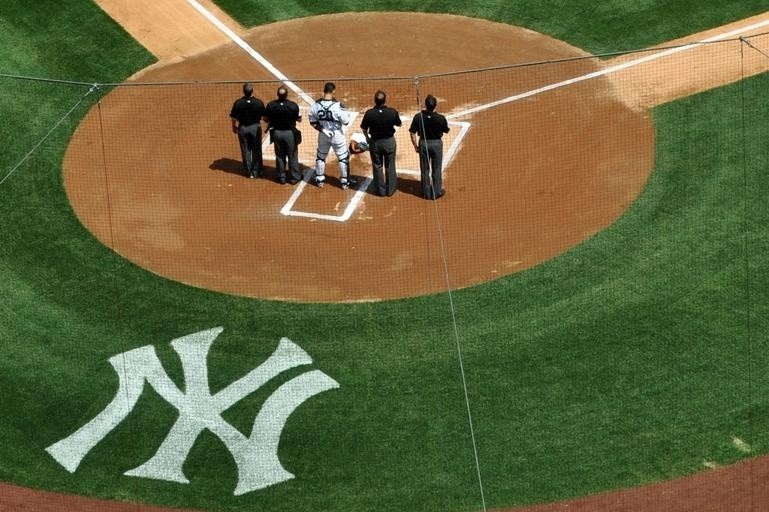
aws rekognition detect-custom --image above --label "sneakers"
[316,174,327,187]
[340,178,358,190]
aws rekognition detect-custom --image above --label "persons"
[409,95,450,200]
[307,82,358,189]
[263,86,304,185]
[360,90,402,197]
[229,82,265,179]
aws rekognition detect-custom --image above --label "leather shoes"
[249,174,304,185]
[426,189,446,200]
